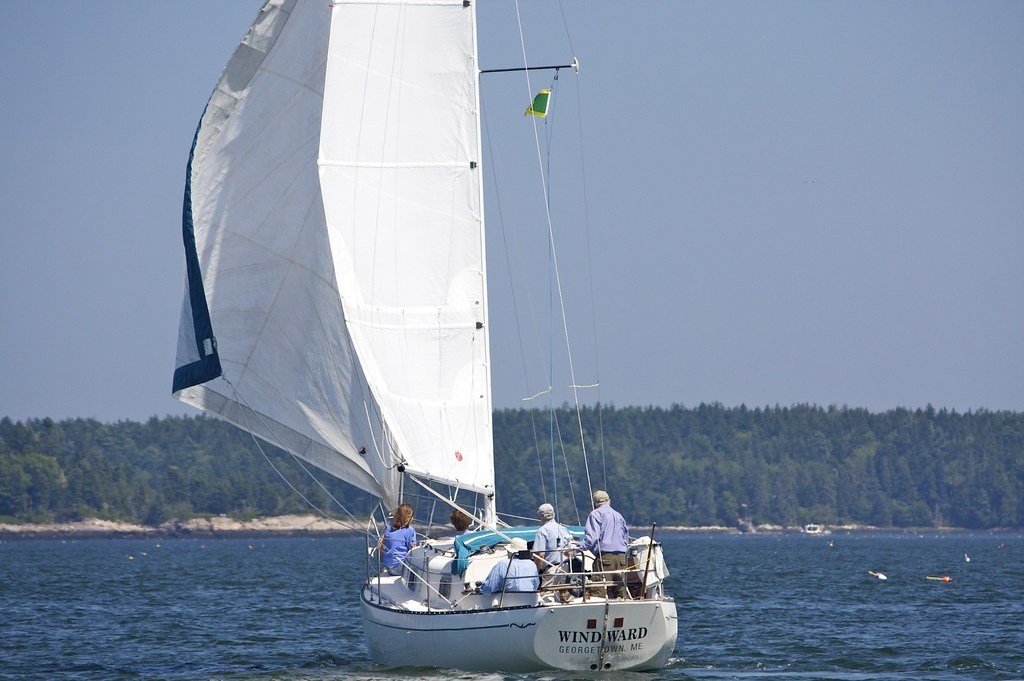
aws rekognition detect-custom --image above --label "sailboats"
[173,0,679,675]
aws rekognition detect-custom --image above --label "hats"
[537,503,555,517]
[504,537,528,555]
[592,490,610,503]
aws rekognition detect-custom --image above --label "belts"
[547,563,560,568]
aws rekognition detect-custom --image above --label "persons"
[376,504,417,576]
[479,537,540,594]
[565,491,629,599]
[532,503,576,590]
[450,509,475,537]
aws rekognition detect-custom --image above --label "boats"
[806,523,831,535]
[926,576,953,582]
[868,570,888,580]
